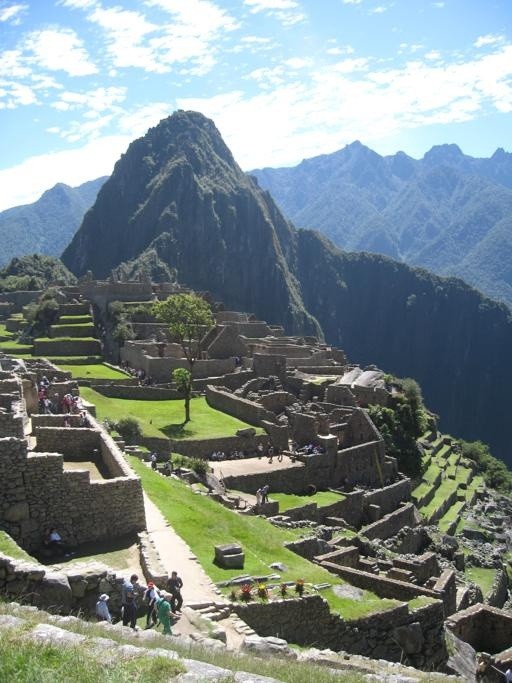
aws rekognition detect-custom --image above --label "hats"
[147,582,153,587]
[99,594,110,601]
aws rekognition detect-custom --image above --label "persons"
[157,594,182,636]
[49,528,75,561]
[95,594,111,623]
[263,483,270,503]
[121,574,140,628]
[64,416,70,427]
[256,486,263,506]
[36,374,77,413]
[43,527,66,554]
[143,582,158,629]
[145,579,160,594]
[164,571,183,614]
[151,452,157,470]
[211,441,324,462]
[79,412,87,426]
